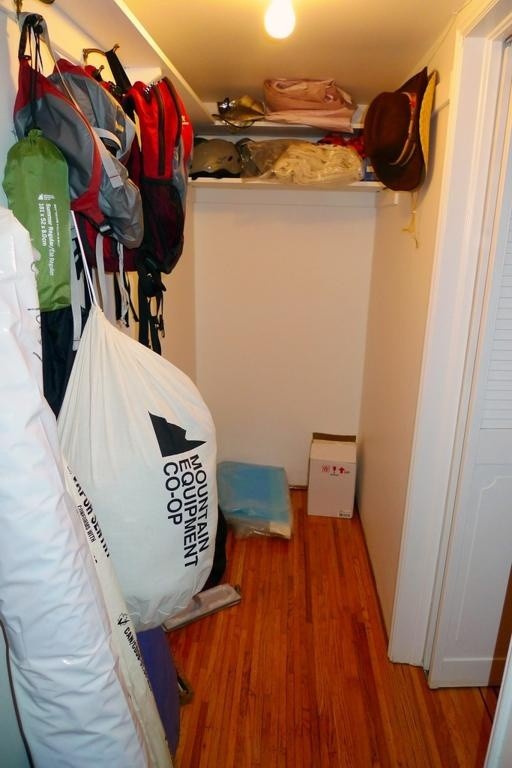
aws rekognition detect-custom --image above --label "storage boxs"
[307,431,357,519]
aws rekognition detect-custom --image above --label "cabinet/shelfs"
[187,118,386,194]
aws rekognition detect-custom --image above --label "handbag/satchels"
[56,307,220,636]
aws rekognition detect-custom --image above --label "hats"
[364,67,437,192]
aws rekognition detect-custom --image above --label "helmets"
[188,138,242,180]
